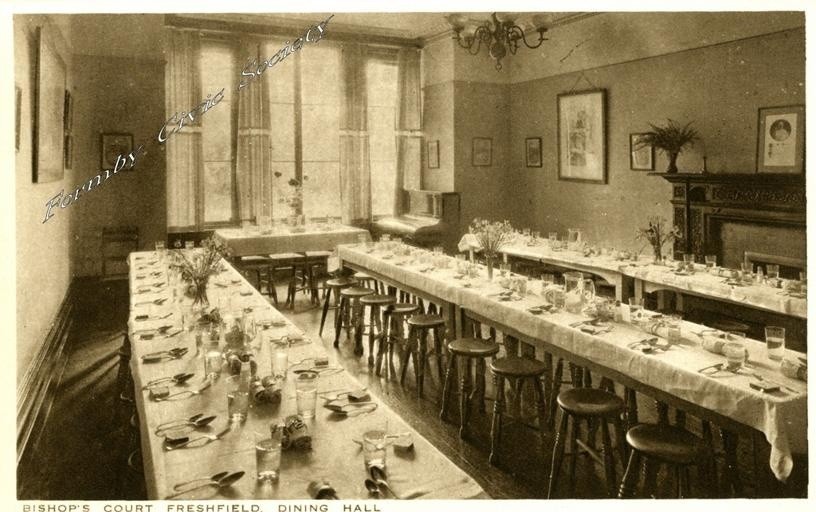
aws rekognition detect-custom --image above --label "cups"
[294,372,318,419]
[240,212,349,235]
[251,421,282,484]
[359,414,389,473]
[523,225,586,259]
[222,376,253,424]
[154,239,194,254]
[499,248,787,385]
[357,231,480,281]
[268,337,289,381]
[314,346,330,367]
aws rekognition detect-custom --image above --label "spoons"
[134,297,245,500]
[370,466,399,499]
[320,386,379,415]
[364,478,385,499]
[299,367,344,379]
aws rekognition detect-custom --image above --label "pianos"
[374,189,460,251]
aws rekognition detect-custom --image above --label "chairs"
[98,223,140,284]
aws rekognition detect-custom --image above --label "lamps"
[441,12,553,72]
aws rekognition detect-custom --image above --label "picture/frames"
[555,87,607,185]
[98,131,134,173]
[753,103,804,175]
[426,139,441,169]
[524,136,542,168]
[14,24,75,187]
[628,132,655,172]
[471,136,492,167]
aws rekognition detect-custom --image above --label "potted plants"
[633,116,703,174]
[631,217,681,264]
[467,216,517,279]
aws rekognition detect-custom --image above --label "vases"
[286,212,308,236]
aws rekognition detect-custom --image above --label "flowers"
[272,168,310,211]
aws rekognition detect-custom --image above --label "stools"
[116,246,806,496]
